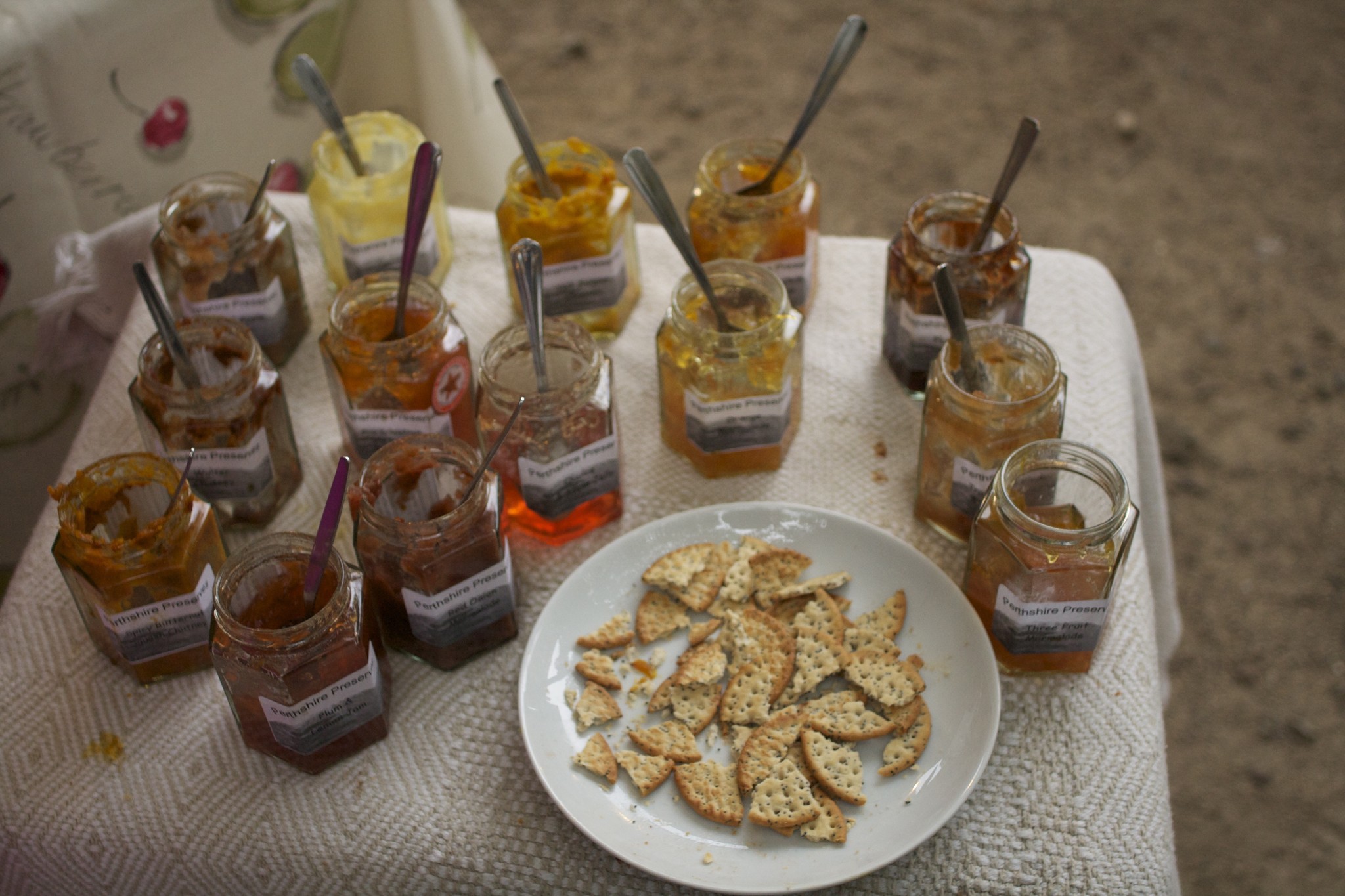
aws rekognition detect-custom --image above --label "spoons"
[621,147,756,358]
[374,142,443,377]
[720,15,869,220]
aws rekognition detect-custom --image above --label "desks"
[0,206,1187,895]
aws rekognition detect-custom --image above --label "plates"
[518,500,1000,896]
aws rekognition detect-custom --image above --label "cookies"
[565,536,931,842]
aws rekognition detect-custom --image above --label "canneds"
[880,191,1139,675]
[660,141,823,475]
[50,111,639,775]
